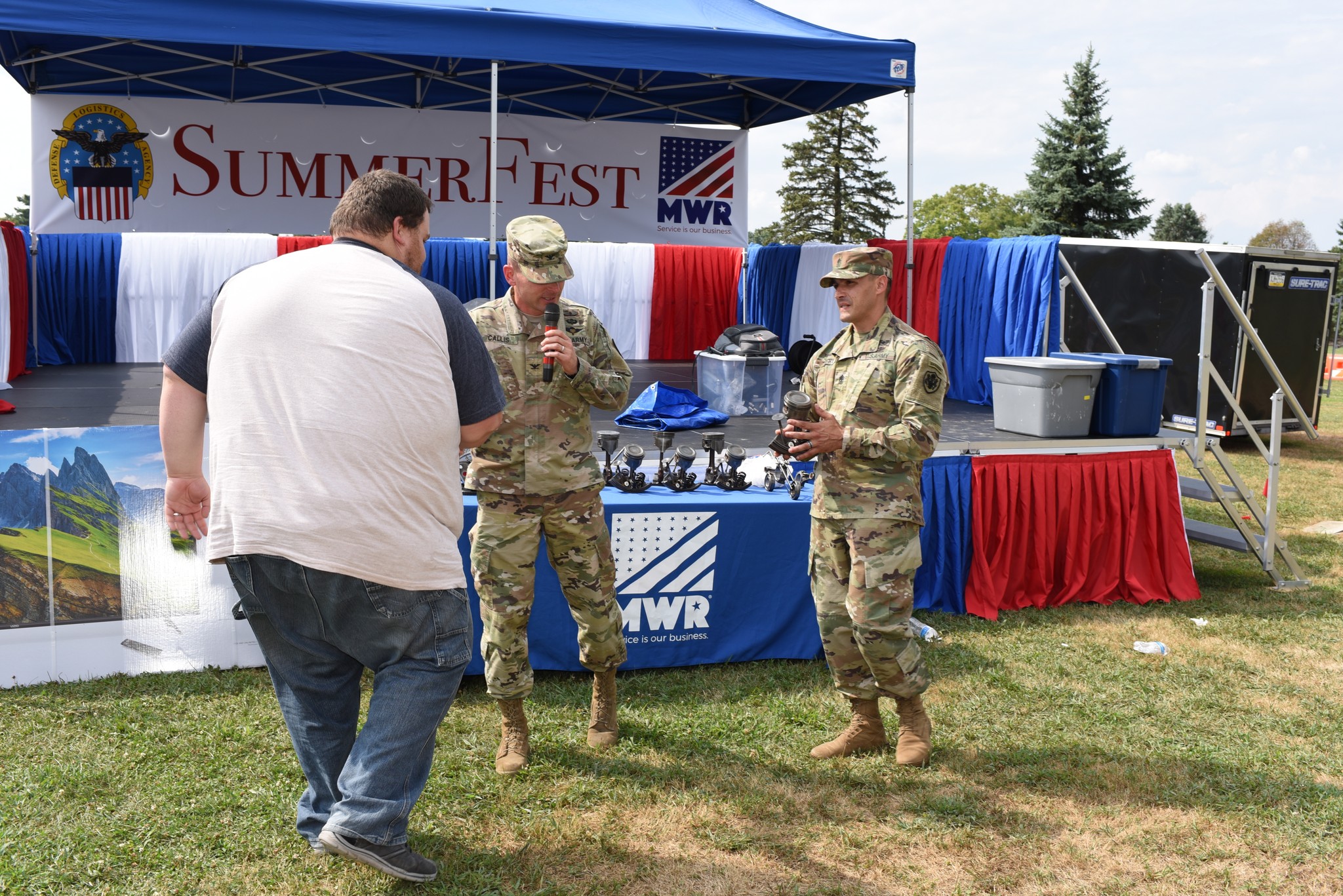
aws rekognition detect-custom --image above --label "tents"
[0,0,918,328]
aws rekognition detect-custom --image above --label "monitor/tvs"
[0,425,200,635]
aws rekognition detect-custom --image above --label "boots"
[587,667,618,749]
[495,697,530,777]
[809,697,890,761]
[893,693,932,767]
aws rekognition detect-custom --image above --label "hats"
[819,247,893,288]
[506,215,575,284]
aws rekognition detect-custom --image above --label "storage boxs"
[984,357,1108,437]
[693,351,788,416]
[1050,352,1173,437]
[1058,238,1341,437]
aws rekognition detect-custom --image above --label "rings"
[807,440,813,449]
[173,513,181,516]
[561,345,564,352]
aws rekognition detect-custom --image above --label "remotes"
[121,639,163,656]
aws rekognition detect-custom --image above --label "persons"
[773,247,950,770]
[468,214,634,777]
[158,168,506,885]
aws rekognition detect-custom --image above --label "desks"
[457,483,825,672]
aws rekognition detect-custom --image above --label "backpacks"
[714,324,786,357]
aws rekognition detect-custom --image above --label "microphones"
[542,303,560,383]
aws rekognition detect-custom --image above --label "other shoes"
[314,830,438,883]
